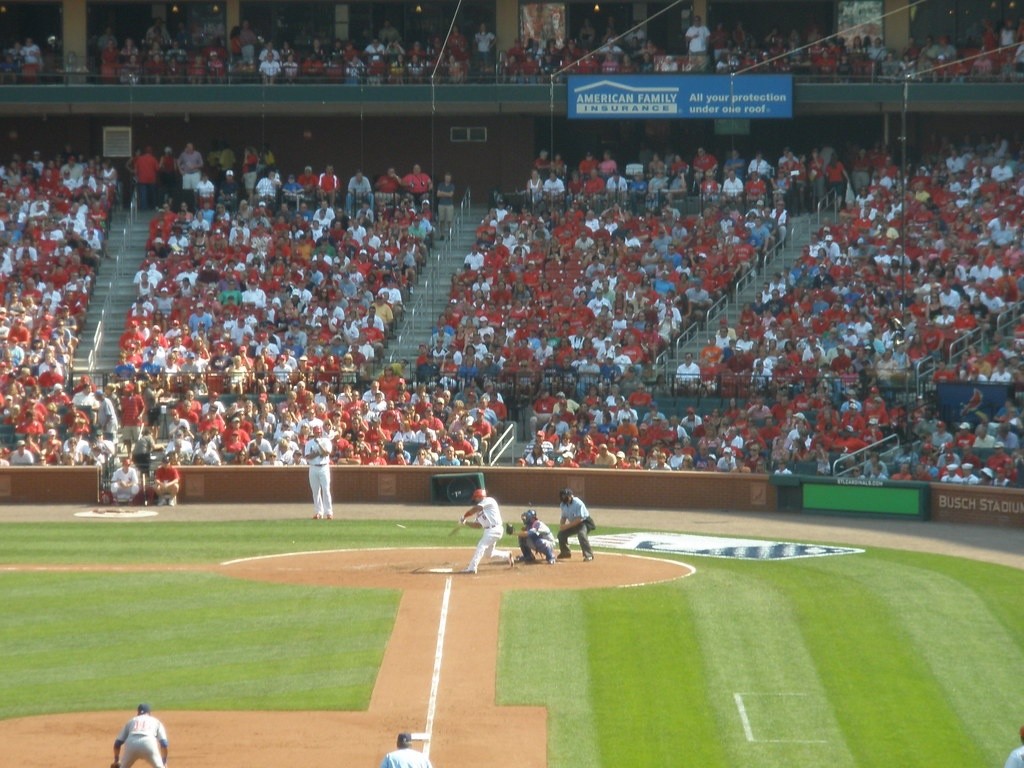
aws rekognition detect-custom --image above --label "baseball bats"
[446,518,466,540]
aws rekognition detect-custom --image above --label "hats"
[469,489,487,501]
[880,245,887,250]
[962,463,974,469]
[209,402,218,412]
[980,467,994,479]
[93,389,104,396]
[937,421,945,428]
[704,415,711,421]
[724,447,732,453]
[137,704,150,714]
[312,425,321,434]
[793,412,806,420]
[846,390,856,395]
[397,732,411,743]
[825,235,833,240]
[958,422,972,430]
[946,463,959,471]
[465,416,474,426]
[622,415,630,422]
[230,416,241,422]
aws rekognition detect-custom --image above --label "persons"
[0,0,1024,488]
[111,702,169,768]
[152,457,180,506]
[111,458,139,506]
[506,509,556,564]
[459,489,515,573]
[557,488,596,562]
[305,425,334,520]
[381,732,433,768]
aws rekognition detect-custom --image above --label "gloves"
[458,516,467,527]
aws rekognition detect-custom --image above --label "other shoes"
[313,513,334,520]
[110,501,119,507]
[548,557,556,565]
[168,498,174,506]
[583,555,594,561]
[158,499,167,506]
[557,552,572,558]
[508,551,515,568]
[127,501,134,508]
[461,568,478,574]
[524,556,536,561]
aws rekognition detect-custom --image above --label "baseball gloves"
[110,763,122,768]
[505,523,515,536]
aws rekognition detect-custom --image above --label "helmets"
[521,509,538,527]
[559,489,575,503]
